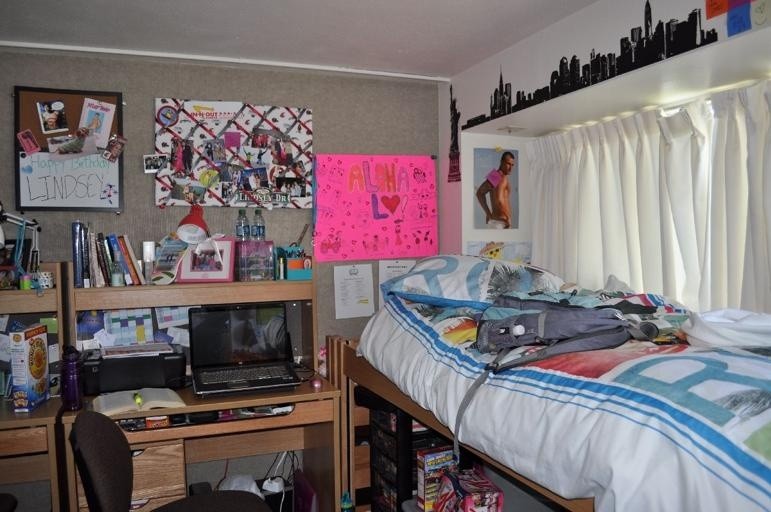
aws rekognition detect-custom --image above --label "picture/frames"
[177,237,235,282]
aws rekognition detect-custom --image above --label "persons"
[477,152,515,229]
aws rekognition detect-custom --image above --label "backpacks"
[475,294,657,376]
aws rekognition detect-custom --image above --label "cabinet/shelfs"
[1,261,318,396]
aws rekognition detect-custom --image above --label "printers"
[82,344,188,395]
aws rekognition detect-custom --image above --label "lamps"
[176,204,211,248]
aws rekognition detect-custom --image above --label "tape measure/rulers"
[296,224,310,246]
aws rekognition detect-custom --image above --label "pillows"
[387,254,566,311]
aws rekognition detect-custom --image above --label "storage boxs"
[415,445,504,512]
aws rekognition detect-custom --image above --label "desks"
[1,362,342,512]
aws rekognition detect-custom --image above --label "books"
[72,221,146,289]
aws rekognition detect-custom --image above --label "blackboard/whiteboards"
[14,86,124,212]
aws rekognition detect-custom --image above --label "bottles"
[251,209,265,240]
[236,209,250,240]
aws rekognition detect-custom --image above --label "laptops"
[188,301,302,394]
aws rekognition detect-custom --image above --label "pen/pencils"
[284,250,305,258]
[134,391,143,406]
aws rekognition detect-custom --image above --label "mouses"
[309,376,325,390]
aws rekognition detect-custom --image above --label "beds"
[324,286,771,512]
